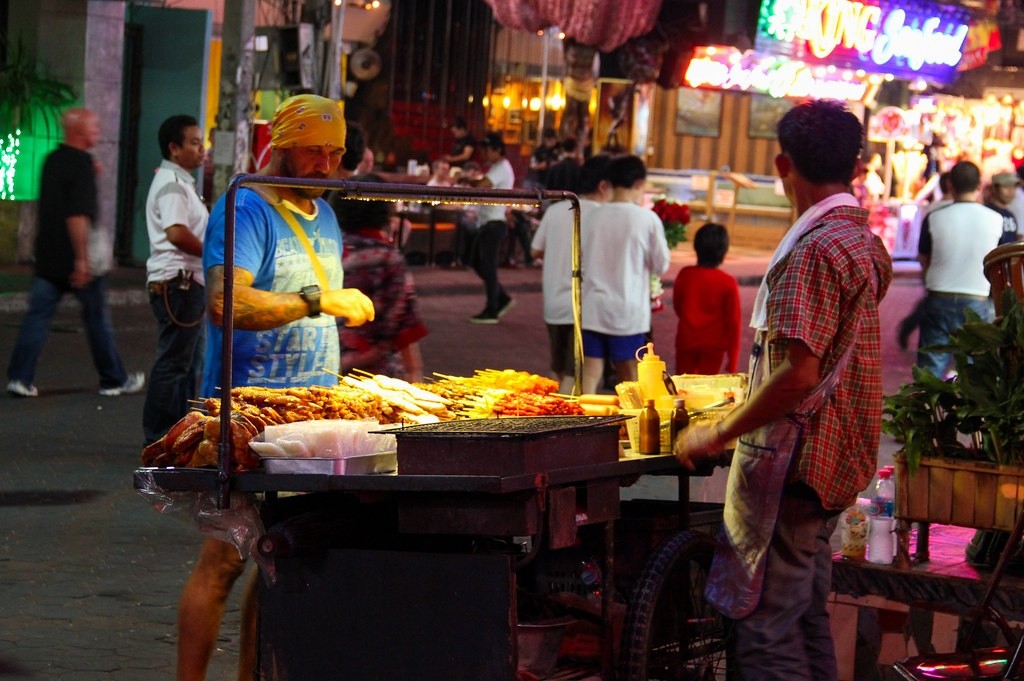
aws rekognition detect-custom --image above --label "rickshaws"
[136,171,751,679]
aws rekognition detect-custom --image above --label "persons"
[893,172,957,351]
[1003,163,1024,237]
[984,172,1017,246]
[670,222,743,375]
[673,98,897,677]
[917,161,1005,393]
[141,112,211,451]
[332,172,433,385]
[6,107,148,400]
[321,116,627,325]
[175,91,379,678]
[527,153,617,395]
[578,155,672,396]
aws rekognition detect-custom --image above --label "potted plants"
[877,285,1024,532]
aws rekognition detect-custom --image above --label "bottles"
[638,399,660,455]
[670,399,689,452]
[882,465,899,557]
[636,343,667,410]
[867,469,894,564]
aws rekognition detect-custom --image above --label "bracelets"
[716,420,728,445]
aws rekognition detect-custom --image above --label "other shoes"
[496,297,514,318]
[468,315,500,325]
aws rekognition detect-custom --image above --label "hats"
[270,94,346,149]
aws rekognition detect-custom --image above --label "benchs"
[821,551,1024,681]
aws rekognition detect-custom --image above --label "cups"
[839,498,870,558]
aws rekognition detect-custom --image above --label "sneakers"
[99,370,146,396]
[7,379,38,396]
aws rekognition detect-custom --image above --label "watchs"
[297,284,322,321]
